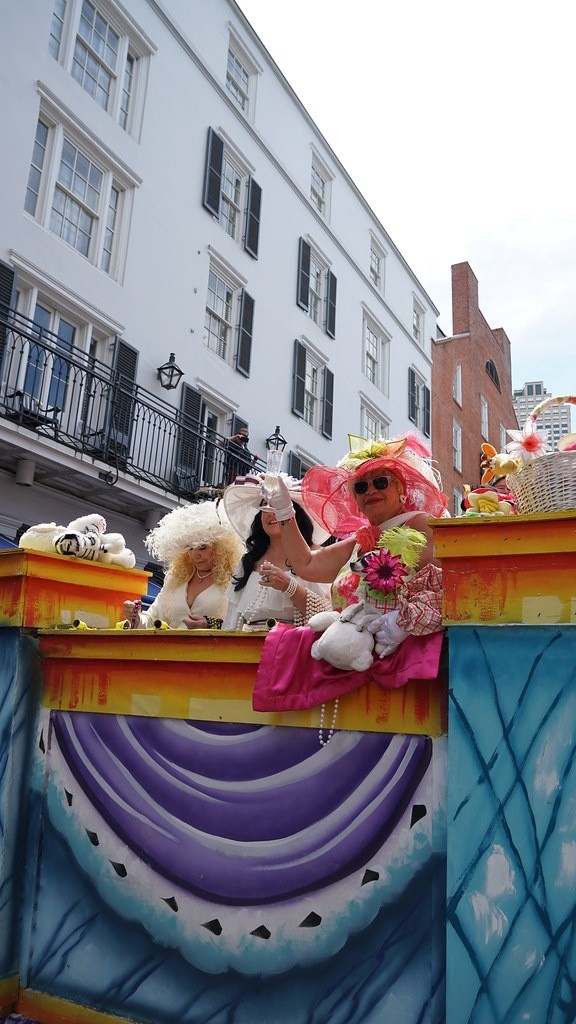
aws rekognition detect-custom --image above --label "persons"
[219,472,332,634]
[490,475,511,495]
[122,501,244,629]
[219,427,258,485]
[261,434,446,659]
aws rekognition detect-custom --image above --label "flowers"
[504,418,553,464]
[362,547,409,598]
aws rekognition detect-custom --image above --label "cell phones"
[240,436,249,443]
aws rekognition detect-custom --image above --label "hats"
[498,493,518,508]
[301,434,451,539]
[460,485,499,512]
[489,474,506,486]
[215,472,338,551]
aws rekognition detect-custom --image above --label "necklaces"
[319,697,339,747]
[197,569,212,583]
[294,587,333,628]
[236,561,273,630]
[130,602,139,629]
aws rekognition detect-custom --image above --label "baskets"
[506,395,576,515]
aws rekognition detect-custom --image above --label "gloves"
[368,610,412,659]
[258,473,296,521]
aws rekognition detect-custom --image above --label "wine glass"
[258,450,284,512]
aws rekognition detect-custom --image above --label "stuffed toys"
[20,513,136,569]
[309,599,387,671]
[480,443,518,484]
[460,488,511,515]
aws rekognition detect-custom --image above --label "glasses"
[352,476,397,496]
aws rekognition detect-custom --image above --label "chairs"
[174,466,206,493]
[78,421,134,471]
[4,384,61,440]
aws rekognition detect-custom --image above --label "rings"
[265,577,269,581]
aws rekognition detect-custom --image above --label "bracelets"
[279,515,295,527]
[282,577,298,597]
[203,616,223,629]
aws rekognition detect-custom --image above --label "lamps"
[156,352,185,390]
[265,425,288,453]
